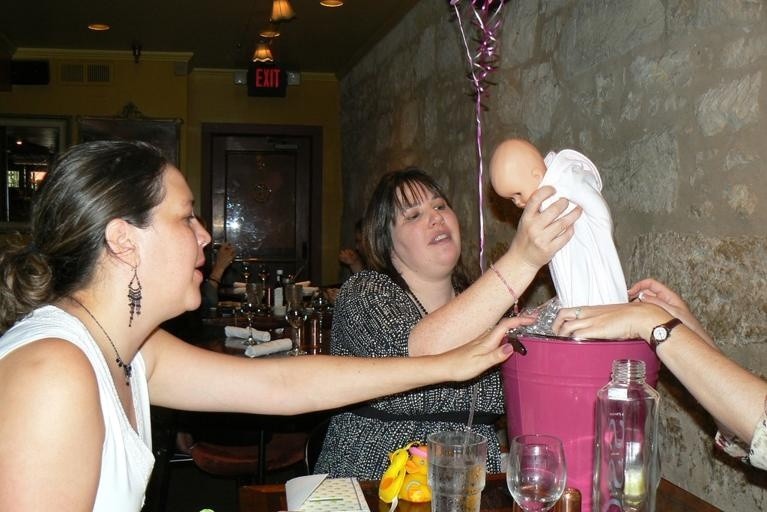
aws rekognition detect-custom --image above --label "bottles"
[590,358,658,512]
[555,489,582,511]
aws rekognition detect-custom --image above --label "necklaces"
[61,293,134,386]
[391,267,430,320]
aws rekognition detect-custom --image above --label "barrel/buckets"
[265,269,296,309]
[497,332,662,512]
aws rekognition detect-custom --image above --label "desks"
[240,473,724,512]
[183,278,340,512]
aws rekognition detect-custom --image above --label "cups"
[609,458,662,512]
[426,429,488,512]
[506,433,567,511]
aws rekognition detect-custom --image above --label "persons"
[303,164,585,480]
[541,274,767,480]
[0,135,541,510]
[334,219,370,283]
[487,137,628,315]
[161,241,237,344]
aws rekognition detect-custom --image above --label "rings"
[487,327,494,331]
[573,305,581,319]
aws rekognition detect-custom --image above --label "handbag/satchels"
[285,473,371,512]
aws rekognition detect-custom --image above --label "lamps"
[250,36,274,64]
[267,0,299,22]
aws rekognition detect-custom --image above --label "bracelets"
[489,264,520,316]
[208,278,222,286]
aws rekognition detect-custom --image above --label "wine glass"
[240,260,328,356]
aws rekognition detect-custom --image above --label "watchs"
[646,317,682,353]
